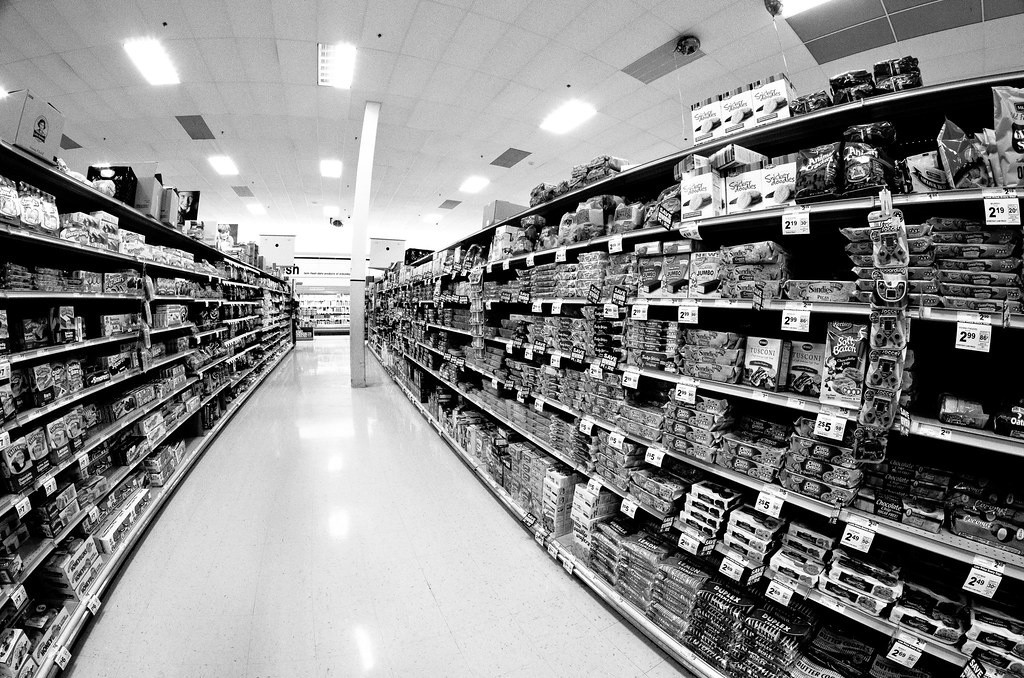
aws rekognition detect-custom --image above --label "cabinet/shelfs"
[360,65,1024,678]
[295,297,350,333]
[0,137,295,678]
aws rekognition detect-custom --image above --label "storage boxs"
[750,72,798,128]
[786,339,826,397]
[491,225,519,263]
[708,143,768,171]
[691,93,728,146]
[673,153,711,182]
[0,87,201,678]
[741,336,792,392]
[482,199,530,229]
[680,173,729,231]
[720,82,758,137]
[725,153,799,215]
